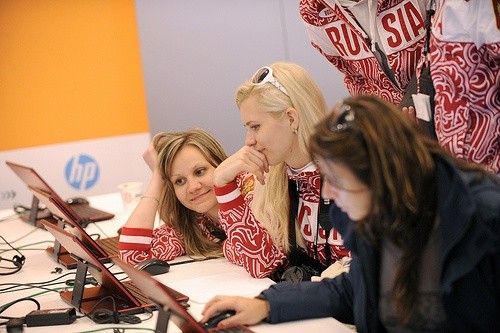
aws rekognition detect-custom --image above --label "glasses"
[328,101,357,132]
[250,66,290,97]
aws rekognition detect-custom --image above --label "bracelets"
[139,196,159,207]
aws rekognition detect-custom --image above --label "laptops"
[5,162,255,333]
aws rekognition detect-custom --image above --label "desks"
[0,193,354,333]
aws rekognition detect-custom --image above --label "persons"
[202,95,500,333]
[300,0,500,175]
[119,129,257,266]
[212,61,352,279]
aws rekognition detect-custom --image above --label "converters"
[25,308,76,328]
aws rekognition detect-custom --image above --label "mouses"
[203,310,235,328]
[134,259,170,276]
[65,199,89,206]
